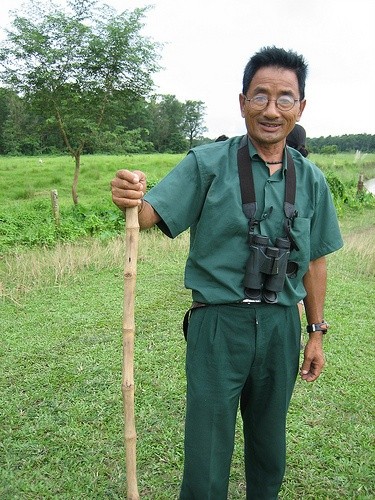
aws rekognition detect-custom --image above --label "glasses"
[244,95,301,112]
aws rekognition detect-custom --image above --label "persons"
[110,46,343,500]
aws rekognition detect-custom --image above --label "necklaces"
[266,161,283,164]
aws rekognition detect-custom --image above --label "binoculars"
[242,230,294,304]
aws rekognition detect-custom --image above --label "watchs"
[306,320,328,334]
